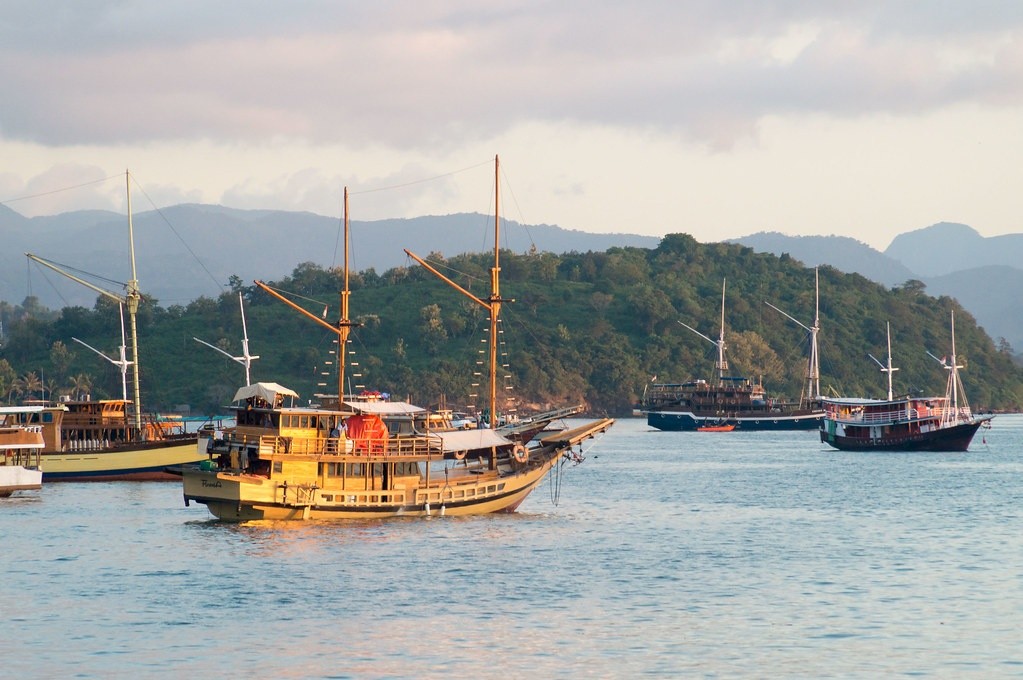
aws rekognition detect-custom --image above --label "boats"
[641,265,846,432]
[818,309,997,451]
[182,155,615,521]
[0,168,236,497]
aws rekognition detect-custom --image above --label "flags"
[651,374,657,382]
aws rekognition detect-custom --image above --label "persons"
[330,419,350,455]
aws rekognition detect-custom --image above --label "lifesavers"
[913,431,919,435]
[894,420,899,426]
[718,399,724,404]
[512,444,529,464]
[463,422,470,430]
[453,450,468,460]
[731,400,736,404]
